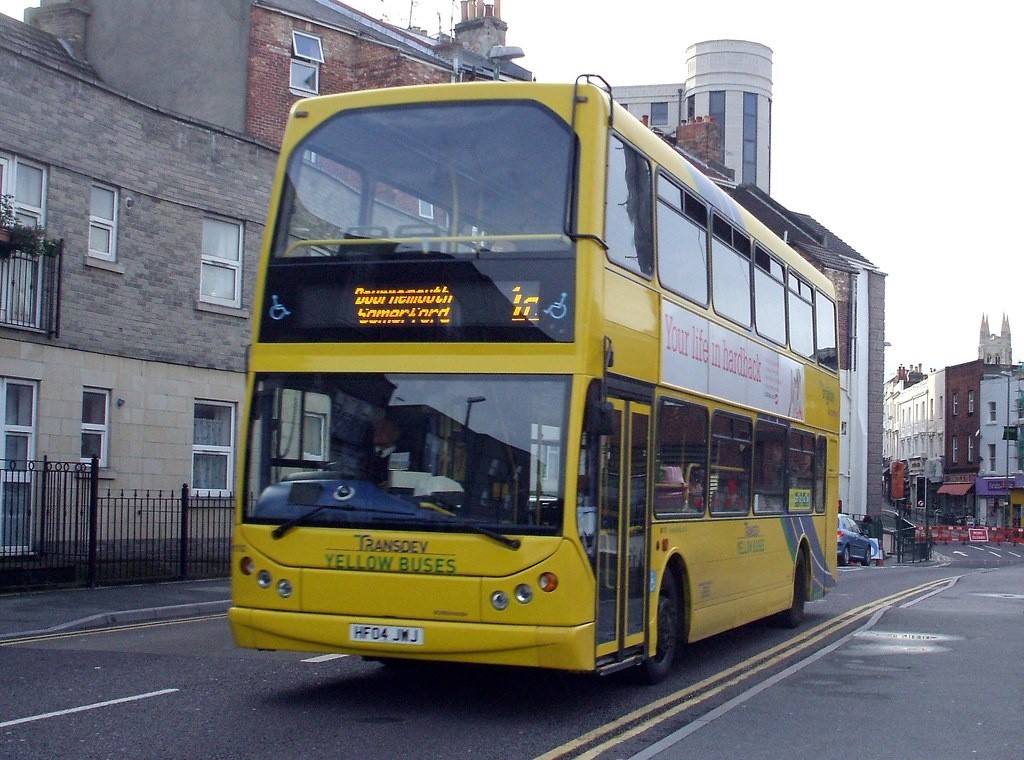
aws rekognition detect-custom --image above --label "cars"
[838,512,873,566]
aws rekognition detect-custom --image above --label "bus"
[223,73,846,691]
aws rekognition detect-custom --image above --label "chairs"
[335,224,442,255]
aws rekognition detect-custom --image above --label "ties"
[373,448,384,472]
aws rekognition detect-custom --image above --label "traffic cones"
[876,544,884,566]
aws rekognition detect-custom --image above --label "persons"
[902,494,912,520]
[341,414,420,494]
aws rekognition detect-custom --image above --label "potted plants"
[0,193,16,243]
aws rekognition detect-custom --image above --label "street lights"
[1000,370,1013,543]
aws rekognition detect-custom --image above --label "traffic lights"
[916,477,926,508]
[903,478,910,497]
